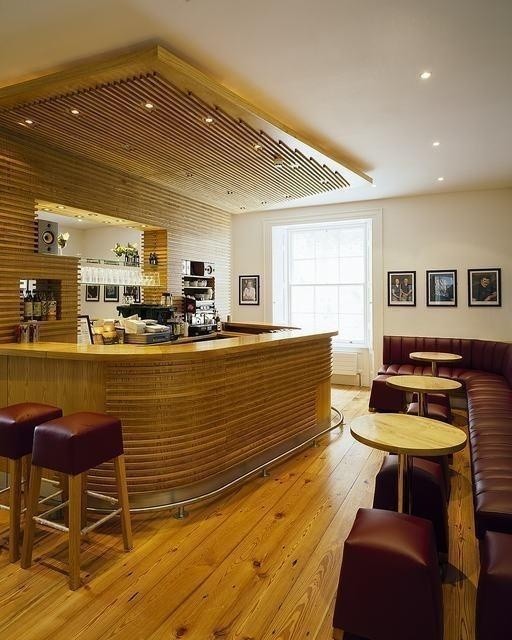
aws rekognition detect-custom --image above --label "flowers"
[113,243,138,257]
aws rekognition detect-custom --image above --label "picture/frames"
[386,271,416,308]
[467,267,502,308]
[103,260,120,303]
[237,275,260,306]
[85,258,100,303]
[426,268,458,308]
[123,261,141,305]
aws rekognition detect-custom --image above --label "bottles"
[216,310,221,332]
[125,249,139,266]
[20,286,57,321]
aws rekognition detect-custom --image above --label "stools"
[0,400,62,563]
[407,402,451,423]
[369,374,407,413]
[372,456,445,521]
[19,410,135,590]
[388,451,453,469]
[473,530,511,640]
[332,508,444,640]
[412,391,450,403]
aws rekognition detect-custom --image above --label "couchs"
[378,335,512,532]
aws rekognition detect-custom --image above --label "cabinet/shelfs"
[182,276,216,326]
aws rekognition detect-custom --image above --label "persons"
[392,278,400,300]
[243,280,255,301]
[401,278,411,301]
[473,274,497,301]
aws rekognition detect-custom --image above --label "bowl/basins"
[184,278,208,287]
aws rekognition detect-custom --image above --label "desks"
[350,412,467,514]
[410,350,463,376]
[386,375,462,417]
